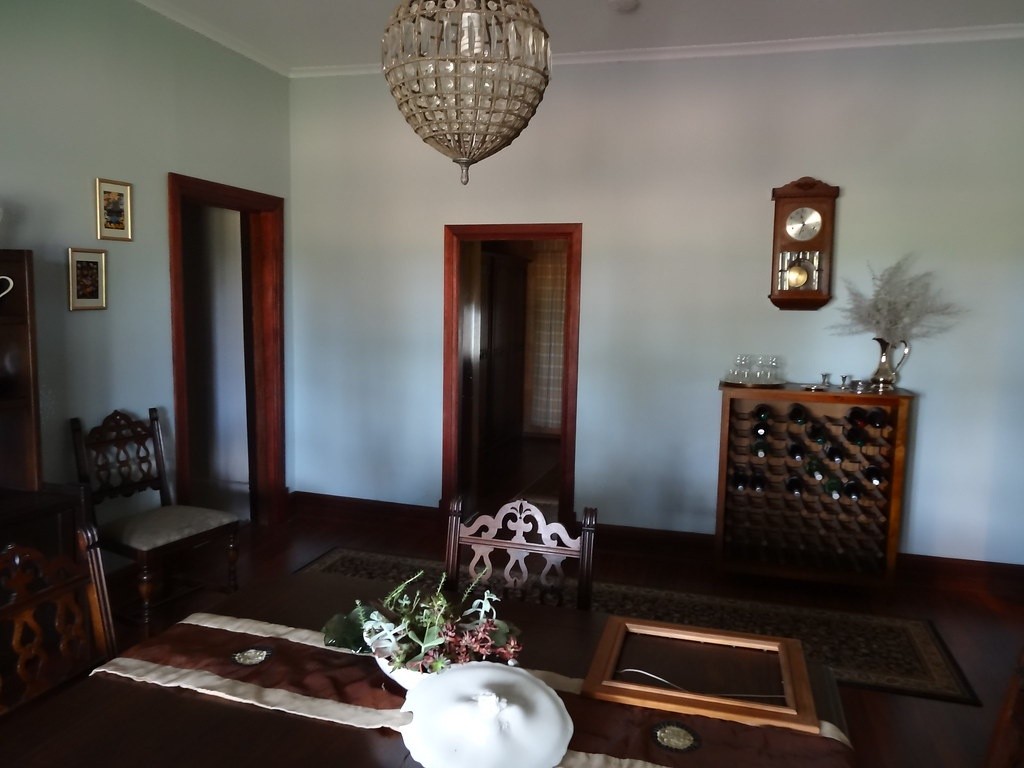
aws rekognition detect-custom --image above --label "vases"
[370,652,574,768]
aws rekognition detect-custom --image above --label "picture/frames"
[69,247,106,310]
[95,177,132,241]
[585,615,821,733]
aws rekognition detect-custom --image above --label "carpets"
[291,547,985,708]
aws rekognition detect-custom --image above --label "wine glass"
[727,353,778,384]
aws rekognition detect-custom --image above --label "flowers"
[322,572,523,671]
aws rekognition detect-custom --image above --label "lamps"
[381,0,552,185]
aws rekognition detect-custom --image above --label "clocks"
[768,176,840,311]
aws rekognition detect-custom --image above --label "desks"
[0,571,856,768]
[0,483,75,557]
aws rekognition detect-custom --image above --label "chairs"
[443,495,599,610]
[68,408,241,640]
[1,524,119,718]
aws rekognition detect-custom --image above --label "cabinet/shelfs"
[0,248,42,492]
[715,380,916,581]
